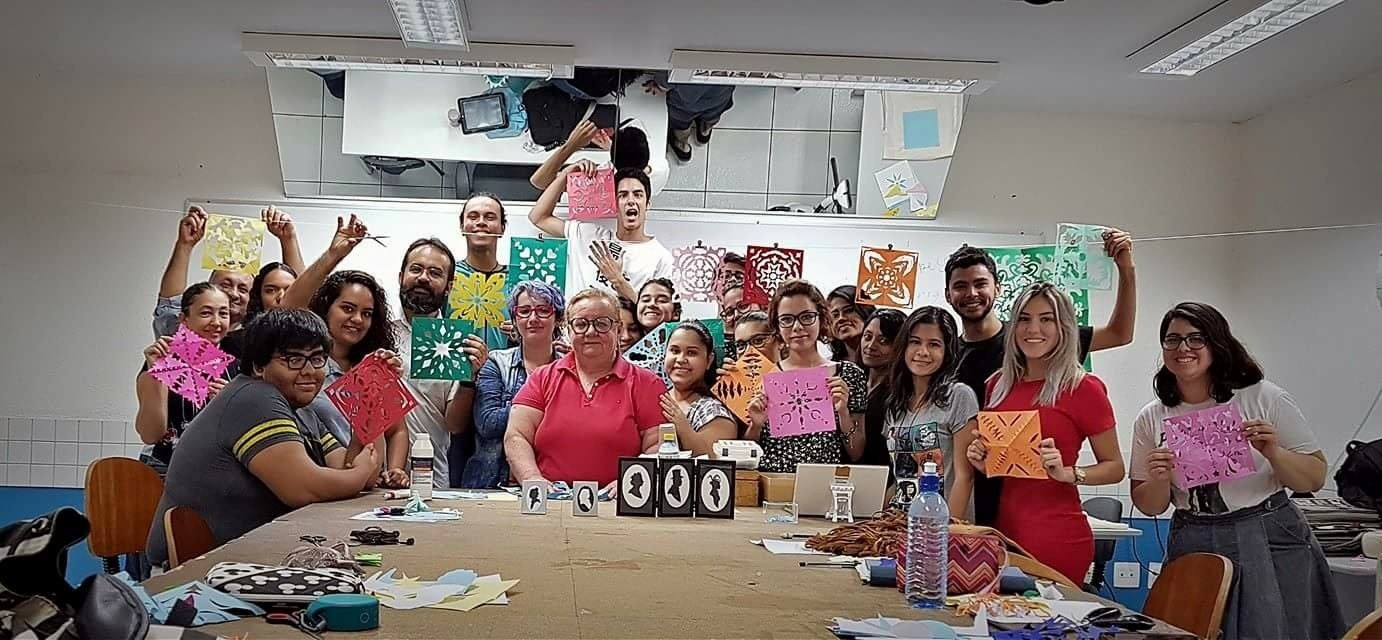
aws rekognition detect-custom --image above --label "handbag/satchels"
[895,531,1002,596]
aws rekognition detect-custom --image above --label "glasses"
[735,337,768,355]
[271,352,328,371]
[568,316,620,334]
[720,300,752,323]
[1161,335,1210,351]
[775,311,820,329]
[408,263,443,280]
[513,305,557,318]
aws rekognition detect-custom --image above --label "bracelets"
[1072,465,1086,485]
[458,380,477,389]
[838,421,861,450]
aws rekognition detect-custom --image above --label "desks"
[88,476,1184,640]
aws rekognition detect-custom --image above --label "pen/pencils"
[798,560,861,568]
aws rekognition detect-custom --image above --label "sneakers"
[667,127,692,164]
[695,117,713,145]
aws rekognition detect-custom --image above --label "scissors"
[1074,606,1157,628]
[300,534,327,549]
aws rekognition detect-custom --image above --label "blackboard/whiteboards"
[183,190,1048,327]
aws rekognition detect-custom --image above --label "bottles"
[658,435,679,458]
[409,432,440,500]
[905,462,948,608]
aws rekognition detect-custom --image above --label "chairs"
[1143,546,1232,640]
[163,506,216,567]
[84,456,167,586]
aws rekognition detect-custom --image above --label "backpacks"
[1333,436,1382,517]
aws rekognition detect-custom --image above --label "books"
[1292,493,1382,556]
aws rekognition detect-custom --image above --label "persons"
[716,311,784,386]
[144,308,403,578]
[461,281,567,490]
[224,261,299,356]
[283,215,411,490]
[503,290,669,499]
[657,318,736,457]
[944,228,1136,522]
[879,306,979,526]
[1129,301,1351,640]
[381,237,489,490]
[711,252,747,319]
[635,278,680,333]
[966,283,1125,591]
[643,71,734,161]
[744,282,868,474]
[823,286,875,373]
[530,121,670,197]
[612,296,642,353]
[450,193,516,349]
[854,307,908,463]
[151,203,306,342]
[720,286,769,327]
[529,158,674,302]
[136,283,242,483]
